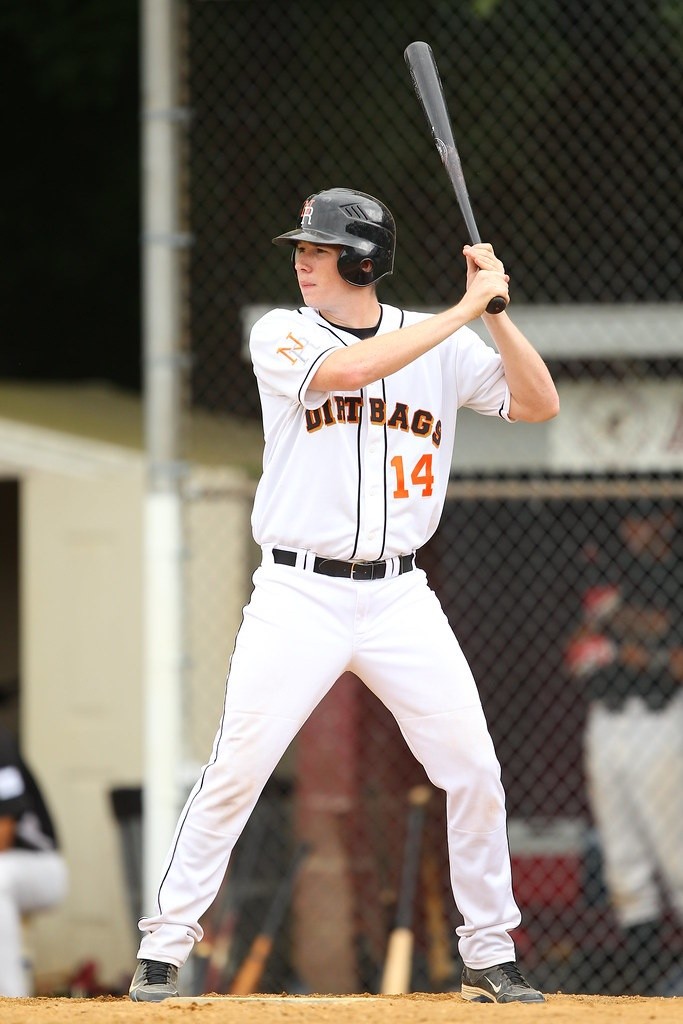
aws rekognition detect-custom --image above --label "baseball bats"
[378,782,430,996]
[400,38,508,316]
[230,840,311,995]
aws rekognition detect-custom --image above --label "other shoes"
[608,921,669,995]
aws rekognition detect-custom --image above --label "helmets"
[0,765,26,818]
[272,187,396,287]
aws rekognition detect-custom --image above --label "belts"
[271,549,414,582]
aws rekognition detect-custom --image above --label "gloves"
[584,662,679,712]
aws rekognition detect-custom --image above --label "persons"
[578,568,683,932]
[129,185,561,1005]
[0,732,72,1003]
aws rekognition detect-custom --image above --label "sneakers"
[128,959,179,1001]
[461,961,548,1005]
